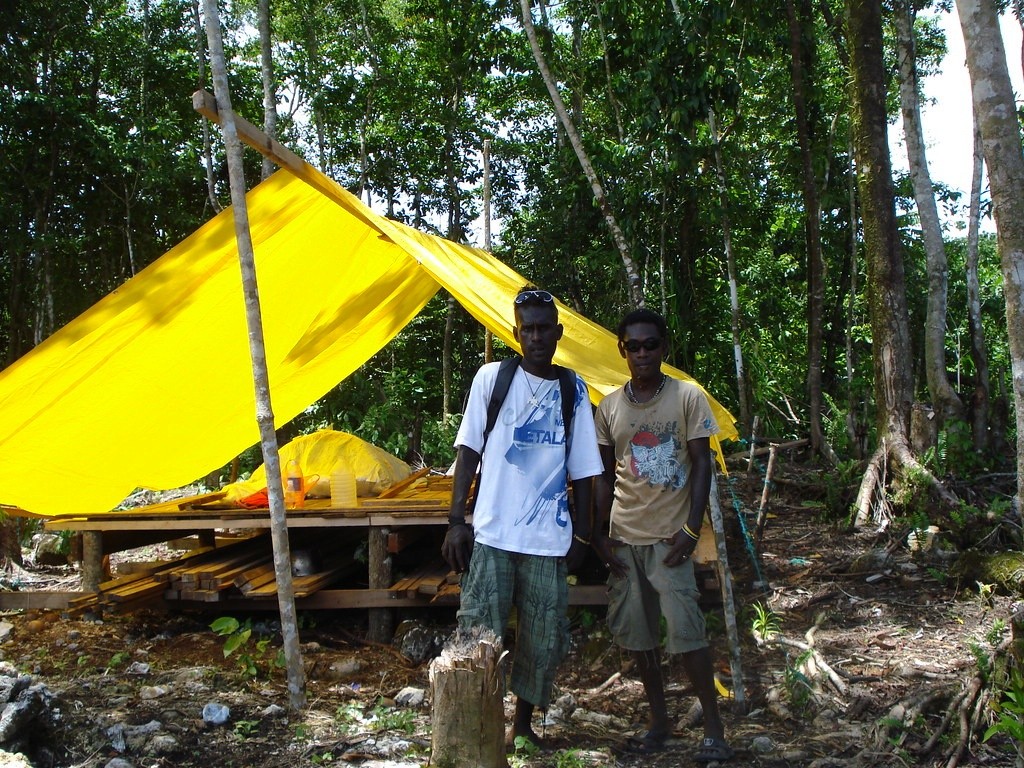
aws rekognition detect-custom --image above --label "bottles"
[286,461,304,508]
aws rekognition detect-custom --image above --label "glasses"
[622,337,664,353]
[514,291,552,303]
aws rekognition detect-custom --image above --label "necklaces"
[629,373,665,403]
[521,362,553,408]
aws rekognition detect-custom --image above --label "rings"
[683,555,688,559]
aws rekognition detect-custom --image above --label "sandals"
[694,737,735,759]
[627,716,674,752]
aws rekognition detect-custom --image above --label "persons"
[593,310,731,760]
[442,285,605,752]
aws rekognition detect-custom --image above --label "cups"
[329,473,357,507]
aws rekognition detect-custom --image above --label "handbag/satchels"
[281,460,320,508]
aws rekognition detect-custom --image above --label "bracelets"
[447,516,466,531]
[573,533,591,545]
[682,523,699,541]
